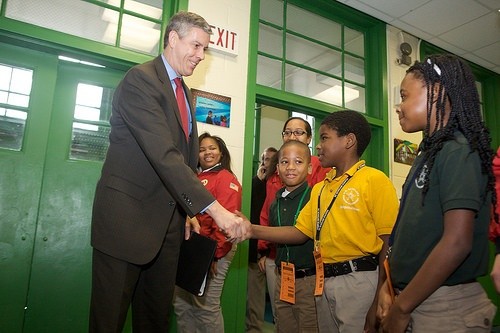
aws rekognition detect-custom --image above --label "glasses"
[281,131,307,137]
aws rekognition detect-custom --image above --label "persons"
[89,11,249,333]
[376,54,500,333]
[218,109,500,333]
[171,132,242,333]
[206,108,229,129]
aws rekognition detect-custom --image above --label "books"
[174,230,217,297]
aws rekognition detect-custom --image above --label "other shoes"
[247,326,261,333]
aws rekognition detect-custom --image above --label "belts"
[277,267,316,279]
[324,254,379,277]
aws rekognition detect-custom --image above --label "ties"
[173,77,189,144]
